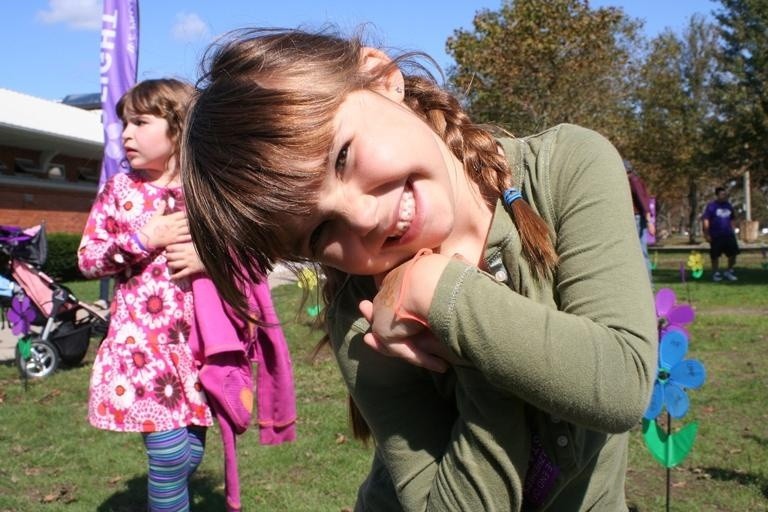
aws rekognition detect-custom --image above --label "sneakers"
[722,271,737,281]
[712,271,722,281]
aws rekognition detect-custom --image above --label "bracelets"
[647,220,651,223]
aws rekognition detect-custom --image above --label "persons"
[184,32,659,512]
[77,78,213,511]
[701,187,740,282]
[622,158,655,289]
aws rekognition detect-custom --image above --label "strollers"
[0,219,112,380]
